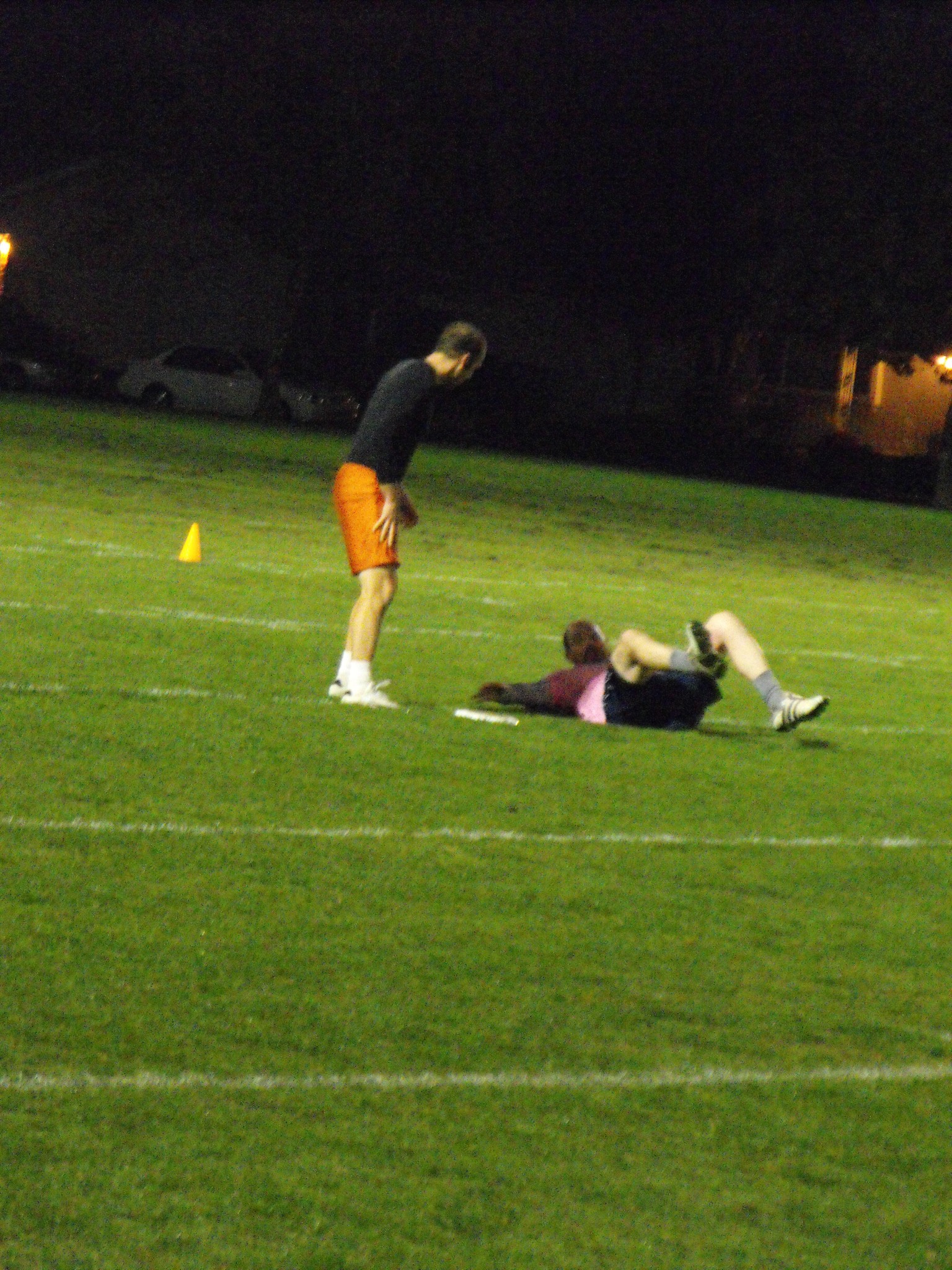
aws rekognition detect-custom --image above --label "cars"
[115,341,275,422]
[0,295,103,382]
[286,374,362,423]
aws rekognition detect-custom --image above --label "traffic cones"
[177,522,201,563]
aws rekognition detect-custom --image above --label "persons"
[330,322,488,707]
[474,611,829,732]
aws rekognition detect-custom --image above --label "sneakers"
[684,620,727,679]
[327,677,397,711]
[771,692,830,733]
